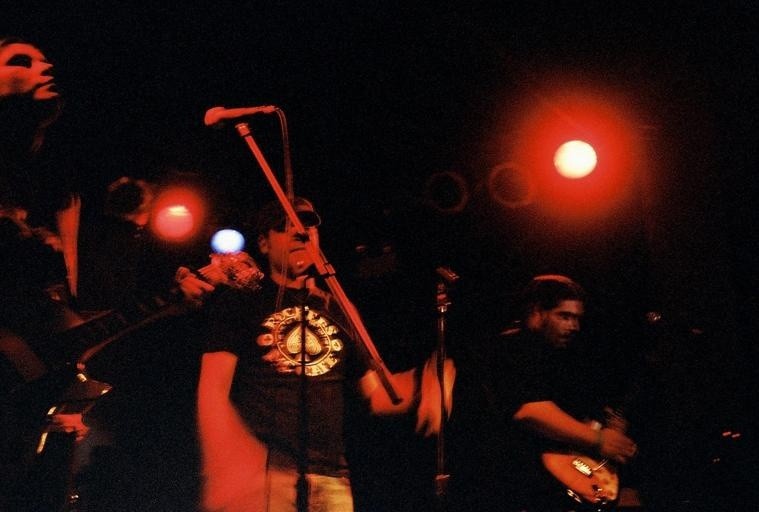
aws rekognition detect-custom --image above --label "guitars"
[0,251,264,463]
[538,404,629,512]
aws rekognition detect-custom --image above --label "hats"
[256,198,320,233]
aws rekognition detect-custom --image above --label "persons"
[0,37,217,512]
[478,275,643,507]
[196,196,456,512]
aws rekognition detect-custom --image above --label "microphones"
[646,311,682,347]
[204,105,277,128]
